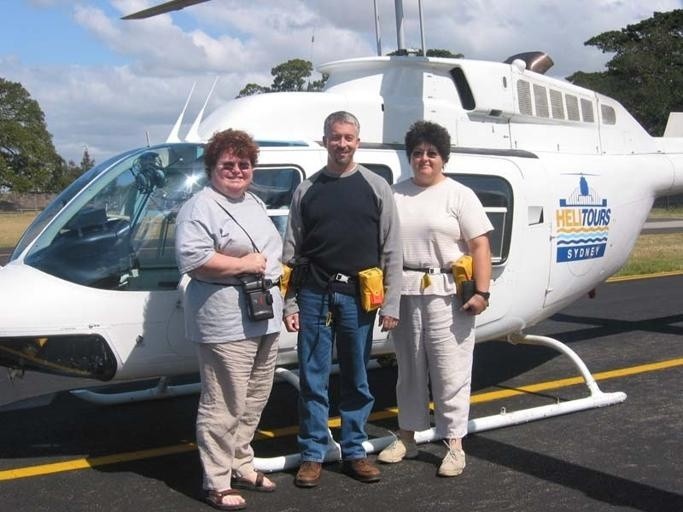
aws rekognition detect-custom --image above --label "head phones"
[135,167,166,191]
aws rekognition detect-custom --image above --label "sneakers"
[436,438,468,479]
[294,460,323,488]
[375,430,419,464]
[341,457,382,483]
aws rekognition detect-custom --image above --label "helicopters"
[0,0,683,475]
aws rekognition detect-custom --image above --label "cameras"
[242,277,274,319]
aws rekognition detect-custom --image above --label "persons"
[278,111,404,486]
[376,120,494,478]
[172,128,285,511]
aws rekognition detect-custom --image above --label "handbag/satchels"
[450,253,476,296]
[277,263,294,299]
[356,264,385,315]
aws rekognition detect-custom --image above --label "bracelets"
[475,290,490,299]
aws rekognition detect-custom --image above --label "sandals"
[230,468,277,493]
[201,488,248,511]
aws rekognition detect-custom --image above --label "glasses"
[215,161,253,171]
[410,149,442,159]
